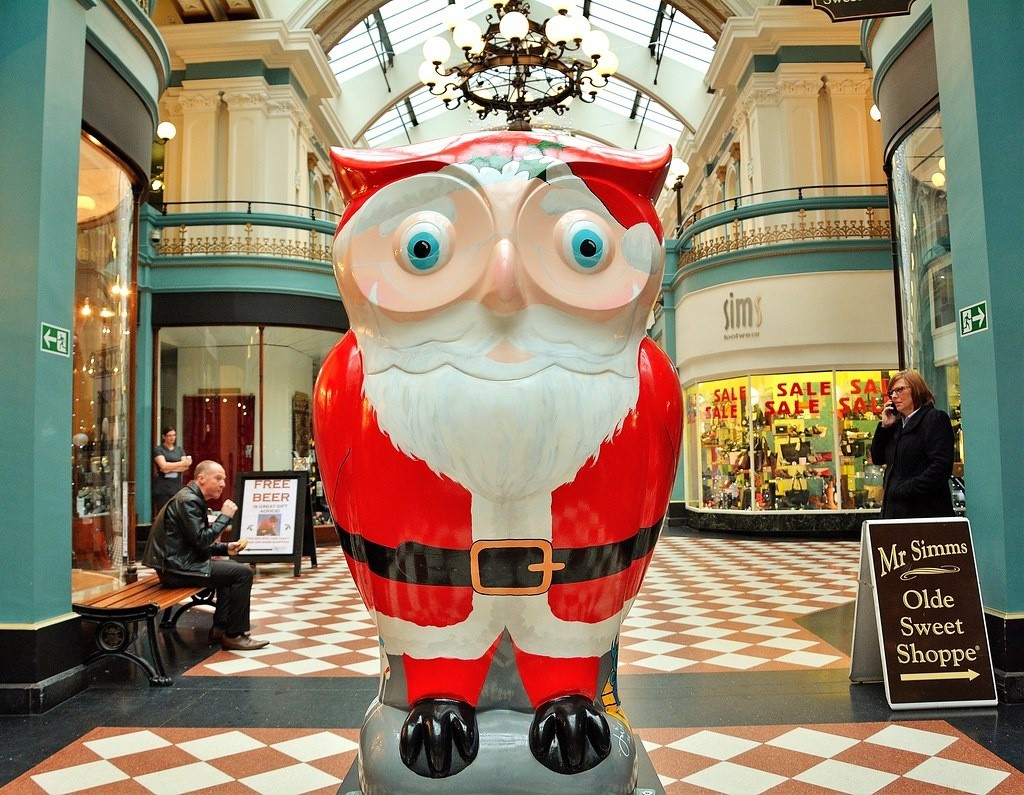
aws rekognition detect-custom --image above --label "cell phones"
[891,402,899,415]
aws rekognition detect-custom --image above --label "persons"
[152,423,192,513]
[142,460,270,651]
[872,370,957,519]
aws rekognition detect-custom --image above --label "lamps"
[665,157,689,237]
[152,121,176,146]
[152,174,164,192]
[420,0,619,131]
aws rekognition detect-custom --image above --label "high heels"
[843,406,858,420]
[804,428,812,437]
[811,495,825,508]
[782,467,793,478]
[795,468,813,477]
[812,426,822,434]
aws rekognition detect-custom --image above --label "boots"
[826,473,856,509]
[769,481,777,509]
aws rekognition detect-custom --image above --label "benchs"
[71,574,218,685]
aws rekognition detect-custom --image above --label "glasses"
[165,434,176,437]
[887,386,911,395]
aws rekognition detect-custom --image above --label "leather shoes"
[208,626,270,650]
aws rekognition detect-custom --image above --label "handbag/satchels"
[780,436,811,464]
[776,426,788,434]
[754,436,777,471]
[734,448,749,469]
[840,438,865,458]
[785,478,810,506]
